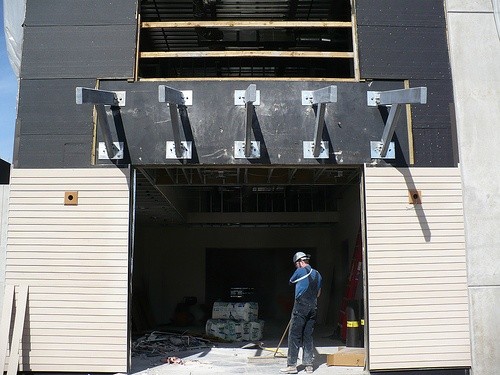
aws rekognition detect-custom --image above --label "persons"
[280,252,322,374]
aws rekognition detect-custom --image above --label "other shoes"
[280,365,298,374]
[305,365,313,373]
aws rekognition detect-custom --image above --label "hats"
[293,251,312,263]
